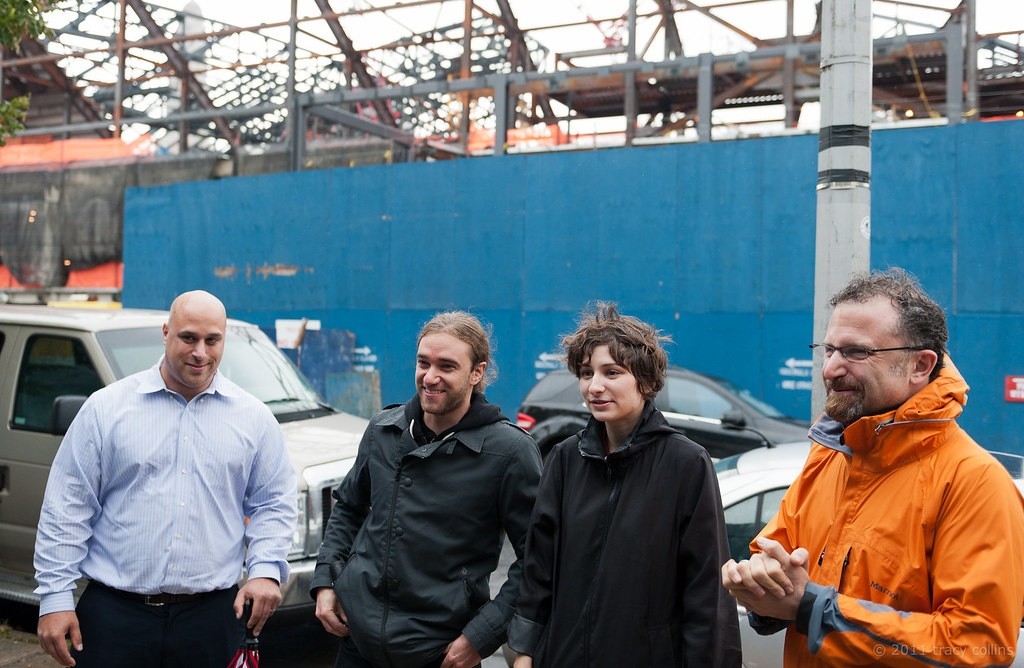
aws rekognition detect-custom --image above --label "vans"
[0,303,370,628]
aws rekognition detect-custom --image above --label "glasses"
[809,342,942,365]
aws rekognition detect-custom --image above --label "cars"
[711,440,1024,668]
[517,365,812,466]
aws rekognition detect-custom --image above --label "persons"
[309,308,544,668]
[721,265,1024,668]
[507,298,743,668]
[33,290,299,668]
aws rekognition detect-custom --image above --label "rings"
[270,609,275,613]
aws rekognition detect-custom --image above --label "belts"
[88,579,239,608]
